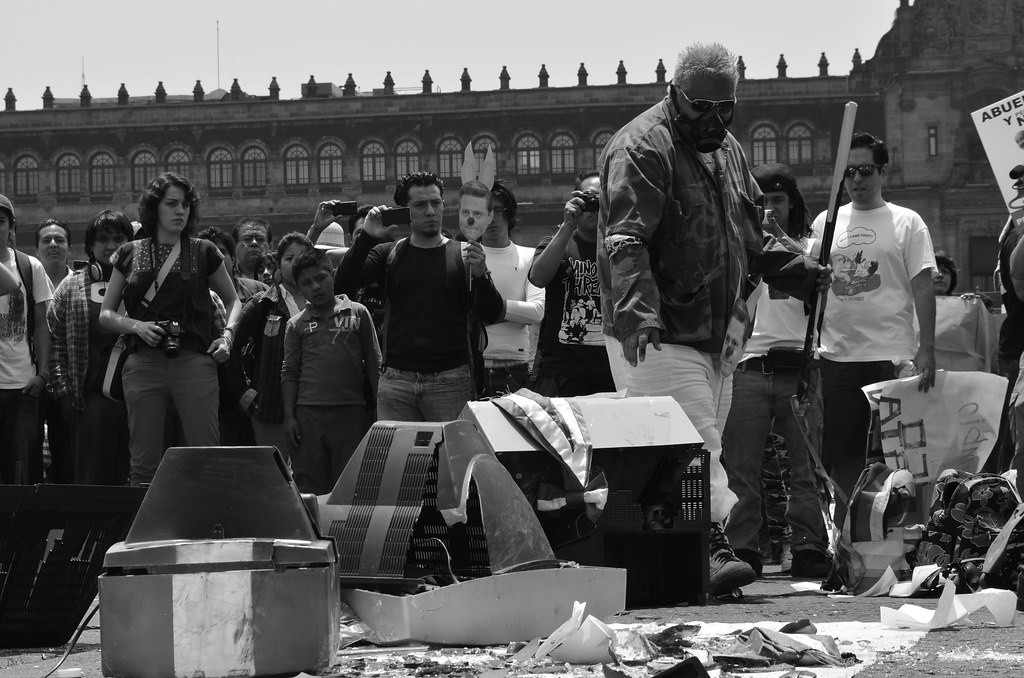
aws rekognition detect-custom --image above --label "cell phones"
[762,209,773,224]
[381,207,411,226]
[332,201,357,216]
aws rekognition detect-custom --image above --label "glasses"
[844,163,880,178]
[493,207,507,215]
[676,84,737,114]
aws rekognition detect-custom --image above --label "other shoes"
[780,551,793,572]
[792,551,830,576]
[821,575,842,592]
[735,550,763,577]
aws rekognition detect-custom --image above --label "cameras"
[152,321,186,359]
[577,194,600,213]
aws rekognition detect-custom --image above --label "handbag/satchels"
[103,338,124,403]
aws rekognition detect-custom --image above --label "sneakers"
[708,523,757,597]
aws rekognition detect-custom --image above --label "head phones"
[88,259,104,282]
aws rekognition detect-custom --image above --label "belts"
[484,358,527,368]
[737,356,774,374]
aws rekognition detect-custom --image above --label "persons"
[99,170,242,486]
[306,172,503,422]
[455,179,546,398]
[27,218,75,484]
[526,44,832,598]
[0,193,57,537]
[195,218,382,496]
[46,209,133,506]
[802,132,1024,590]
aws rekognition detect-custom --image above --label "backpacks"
[839,462,917,597]
[916,467,1024,598]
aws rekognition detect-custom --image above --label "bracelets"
[220,327,235,350]
[38,370,51,384]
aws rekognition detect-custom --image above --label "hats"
[306,222,345,249]
[750,163,799,193]
[0,194,14,214]
